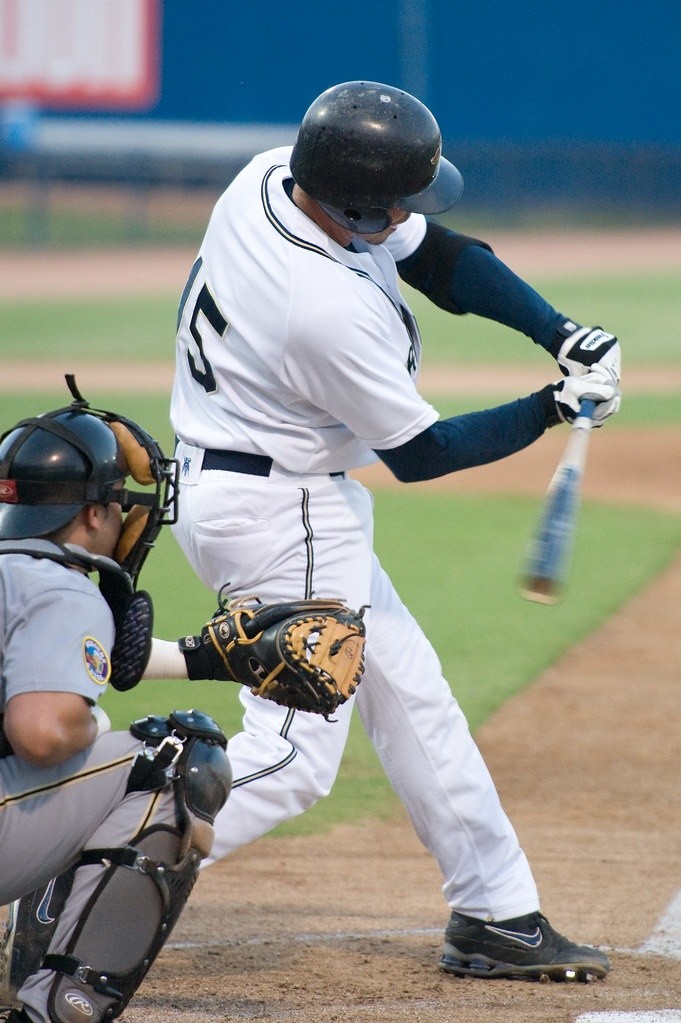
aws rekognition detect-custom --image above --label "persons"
[0,404,364,1023]
[167,79,620,982]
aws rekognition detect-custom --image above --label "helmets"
[1,403,183,542]
[291,80,465,236]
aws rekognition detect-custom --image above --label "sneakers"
[439,911,611,985]
[2,875,72,997]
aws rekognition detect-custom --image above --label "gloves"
[555,328,625,429]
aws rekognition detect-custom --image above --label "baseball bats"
[518,402,596,603]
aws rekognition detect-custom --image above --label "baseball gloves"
[204,596,367,714]
